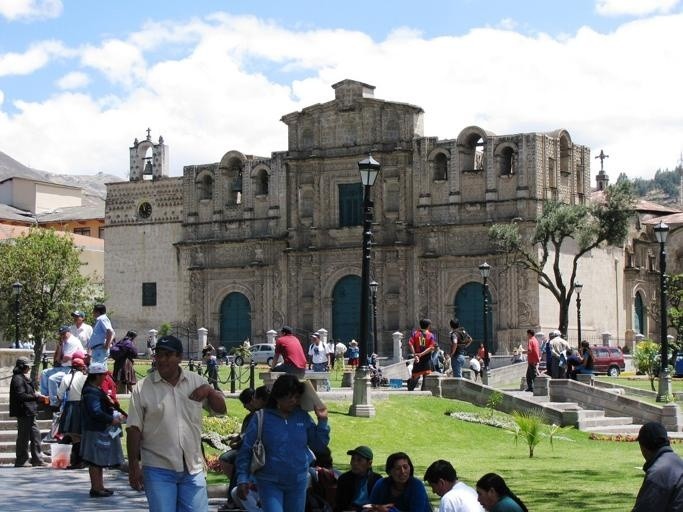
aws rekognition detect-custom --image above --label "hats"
[58,327,70,334]
[636,422,669,446]
[347,446,373,460]
[349,340,358,346]
[549,332,554,337]
[553,330,562,336]
[16,357,36,366]
[71,310,85,318]
[89,363,109,374]
[152,336,183,353]
[310,332,321,338]
[72,358,87,367]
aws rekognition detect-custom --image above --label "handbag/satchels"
[584,356,593,370]
[252,442,266,473]
[51,411,65,439]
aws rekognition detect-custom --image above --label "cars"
[231,343,284,366]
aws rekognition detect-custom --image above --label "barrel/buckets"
[50,442,74,468]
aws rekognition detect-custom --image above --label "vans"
[653,342,682,377]
[577,346,625,376]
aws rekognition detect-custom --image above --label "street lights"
[574,280,583,345]
[653,221,675,402]
[11,278,22,349]
[478,262,491,370]
[348,154,376,418]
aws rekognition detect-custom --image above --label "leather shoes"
[90,489,112,498]
[32,463,47,467]
[103,489,114,494]
[14,462,33,467]
[525,388,533,392]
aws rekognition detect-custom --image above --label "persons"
[511,330,593,391]
[328,339,359,369]
[308,333,330,391]
[9,304,228,496]
[236,375,330,511]
[406,319,487,391]
[244,337,250,347]
[220,386,342,510]
[125,336,226,512]
[338,446,527,512]
[632,422,682,511]
[269,326,307,373]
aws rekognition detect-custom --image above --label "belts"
[91,343,103,350]
